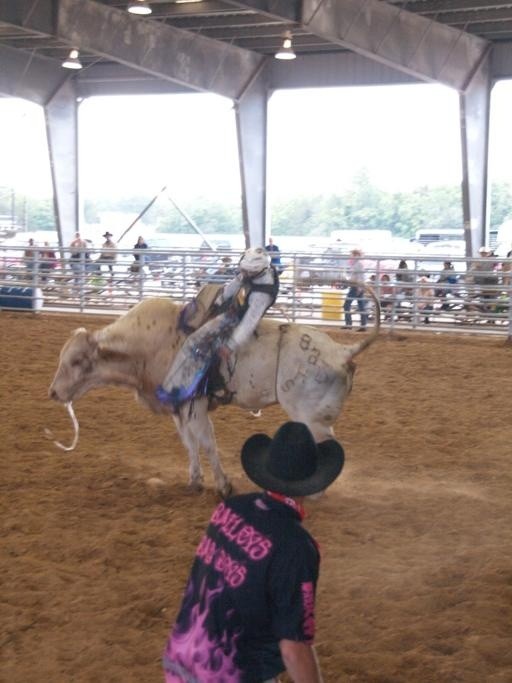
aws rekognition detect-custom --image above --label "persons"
[132,237,149,266]
[210,256,239,285]
[153,245,280,409]
[473,247,512,312]
[156,417,347,683]
[69,233,92,266]
[342,251,368,332]
[26,242,56,280]
[368,260,458,325]
[99,232,118,275]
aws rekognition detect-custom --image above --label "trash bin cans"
[322,292,343,320]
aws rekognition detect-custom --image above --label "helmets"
[239,246,272,273]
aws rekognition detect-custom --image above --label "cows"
[47,276,381,500]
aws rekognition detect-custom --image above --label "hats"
[102,232,112,236]
[241,421,344,496]
